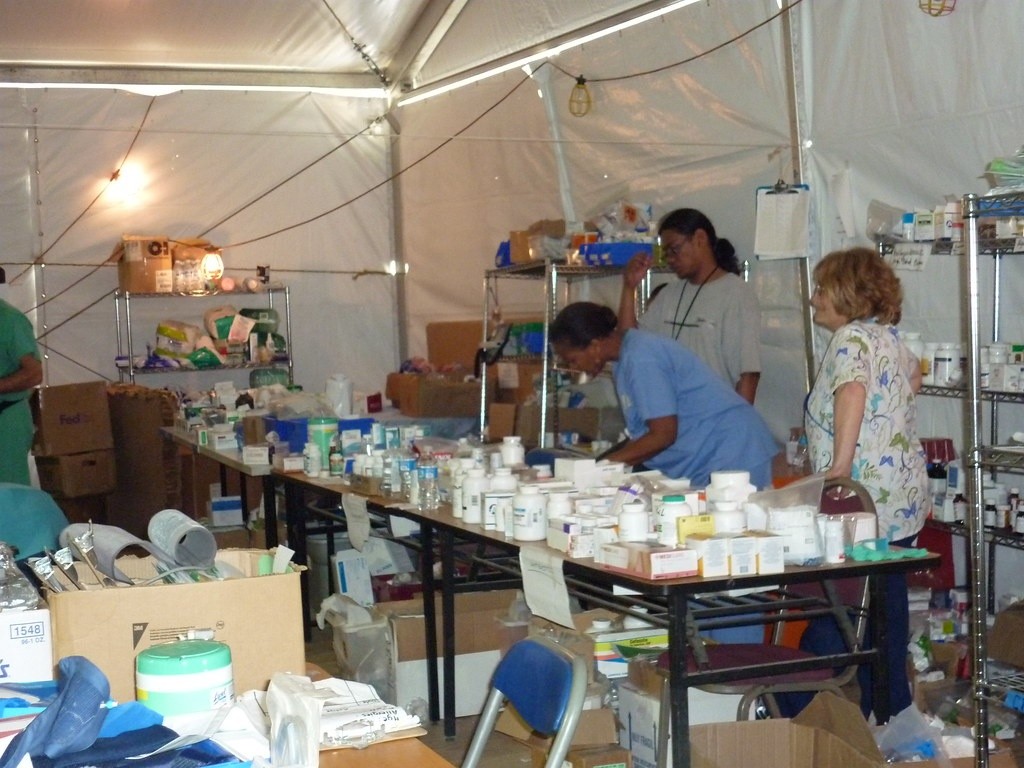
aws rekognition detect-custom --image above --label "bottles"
[927,456,947,494]
[302,372,438,511]
[902,213,913,241]
[786,431,809,468]
[214,276,262,291]
[898,330,1024,391]
[983,488,1024,536]
[234,385,271,411]
[449,436,757,541]
[818,516,845,563]
[881,736,937,763]
[953,491,968,526]
[135,638,233,715]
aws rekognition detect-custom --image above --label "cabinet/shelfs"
[551,261,752,447]
[966,190,1024,768]
[114,287,295,391]
[875,237,1004,612]
[480,257,571,450]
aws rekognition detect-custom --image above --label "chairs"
[652,476,881,768]
[461,632,589,768]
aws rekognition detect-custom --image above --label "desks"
[270,465,547,725]
[305,662,459,768]
[401,501,946,768]
[161,423,281,549]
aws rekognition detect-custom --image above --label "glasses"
[661,236,689,256]
[813,283,829,296]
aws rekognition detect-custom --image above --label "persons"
[0,265,44,487]
[616,206,762,405]
[759,247,932,723]
[548,303,781,495]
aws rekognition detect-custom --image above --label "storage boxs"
[0,589,53,685]
[193,426,209,446]
[399,376,479,421]
[208,429,239,450]
[384,372,466,410]
[597,537,697,582]
[585,243,654,266]
[744,528,787,576]
[207,494,244,526]
[37,450,115,499]
[716,530,757,576]
[544,517,580,554]
[267,413,375,454]
[490,400,517,438]
[349,598,883,768]
[570,233,600,254]
[569,532,594,559]
[479,491,515,533]
[110,395,164,500]
[519,405,599,447]
[48,549,306,704]
[57,495,105,525]
[176,418,200,434]
[607,481,643,514]
[180,448,222,518]
[242,442,272,464]
[112,234,171,292]
[686,533,729,578]
[508,229,543,262]
[197,517,251,550]
[250,521,287,548]
[31,379,114,455]
[242,414,266,444]
[104,493,165,536]
[274,452,304,473]
